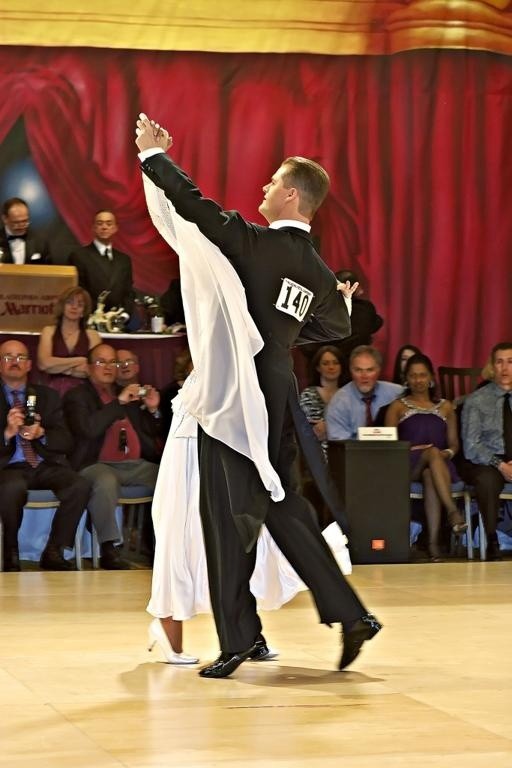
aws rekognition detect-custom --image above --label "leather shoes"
[338,615,382,670]
[39,550,77,571]
[99,555,129,570]
[3,548,20,572]
[428,522,502,562]
[198,632,269,678]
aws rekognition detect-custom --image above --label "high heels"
[147,617,198,663]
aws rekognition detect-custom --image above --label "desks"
[0,330,184,339]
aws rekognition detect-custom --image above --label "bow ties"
[7,234,26,241]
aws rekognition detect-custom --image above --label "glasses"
[95,359,118,368]
[118,359,135,368]
[3,355,30,363]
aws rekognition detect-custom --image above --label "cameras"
[24,387,43,426]
[120,430,127,451]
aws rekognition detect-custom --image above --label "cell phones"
[137,387,147,397]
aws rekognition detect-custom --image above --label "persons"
[0,287,190,571]
[142,120,359,664]
[66,210,133,312]
[135,112,383,678]
[301,270,512,561]
[161,279,186,333]
[1,198,52,264]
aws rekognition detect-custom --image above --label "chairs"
[410,367,512,561]
[25,485,151,570]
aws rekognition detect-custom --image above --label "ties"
[104,248,109,259]
[365,398,373,426]
[10,390,39,468]
[503,394,512,463]
